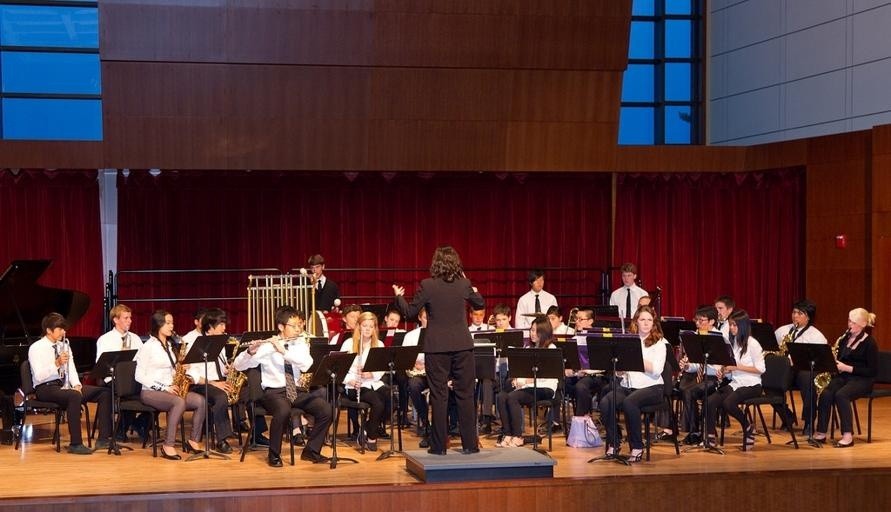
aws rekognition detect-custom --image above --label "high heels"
[627,449,645,462]
[607,442,621,457]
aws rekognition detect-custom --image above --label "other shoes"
[719,419,729,426]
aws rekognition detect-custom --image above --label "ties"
[282,344,298,404]
[535,296,541,319]
[792,329,798,339]
[626,289,632,320]
[150,334,176,369]
[51,344,66,381]
[717,322,724,330]
[214,358,224,381]
[315,280,323,305]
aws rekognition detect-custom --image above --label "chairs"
[1,344,891,466]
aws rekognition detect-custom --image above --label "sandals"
[745,426,756,450]
[708,433,717,447]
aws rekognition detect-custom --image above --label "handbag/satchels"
[567,414,603,447]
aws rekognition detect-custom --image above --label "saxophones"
[221,337,248,405]
[761,325,797,357]
[171,330,194,398]
[814,327,852,390]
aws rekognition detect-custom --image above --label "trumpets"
[59,331,74,391]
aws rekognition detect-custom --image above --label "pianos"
[0,258,98,445]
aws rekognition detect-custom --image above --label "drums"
[309,309,345,342]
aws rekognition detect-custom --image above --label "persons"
[467,305,610,447]
[609,264,649,321]
[300,254,336,318]
[766,300,879,446]
[330,304,457,451]
[95,304,333,467]
[599,296,766,462]
[393,248,485,455]
[28,314,121,452]
[514,270,558,338]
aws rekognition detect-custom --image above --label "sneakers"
[496,437,525,448]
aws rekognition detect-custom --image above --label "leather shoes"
[539,421,561,434]
[678,432,701,445]
[808,435,827,443]
[836,438,854,448]
[464,447,480,454]
[653,430,673,442]
[67,410,432,467]
[428,446,447,455]
[803,422,813,436]
[780,414,797,432]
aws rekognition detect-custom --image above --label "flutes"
[239,333,304,347]
